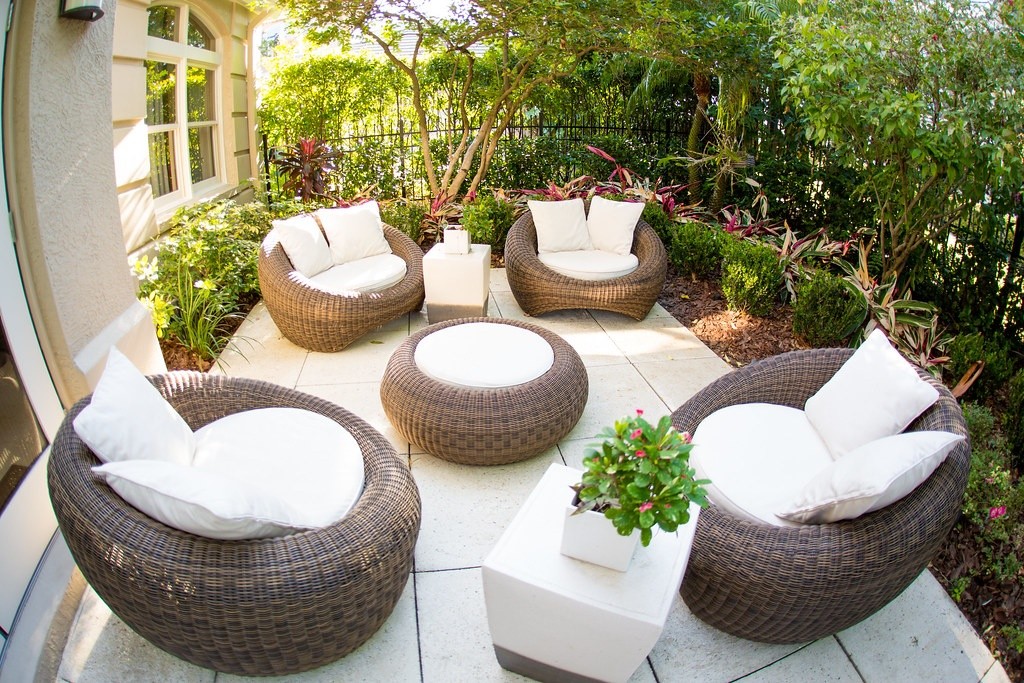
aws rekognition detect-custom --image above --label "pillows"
[587,196,645,255]
[804,329,938,460]
[74,345,198,464]
[90,462,322,541]
[526,199,592,253]
[317,201,393,266]
[775,432,964,525]
[269,215,334,278]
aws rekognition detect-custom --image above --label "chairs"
[666,348,970,644]
[258,209,426,353]
[46,371,421,676]
[505,200,667,322]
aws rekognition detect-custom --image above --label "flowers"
[566,410,711,546]
[131,208,263,376]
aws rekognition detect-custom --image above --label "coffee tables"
[381,317,587,466]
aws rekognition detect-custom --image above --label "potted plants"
[444,195,512,254]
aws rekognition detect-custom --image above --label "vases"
[557,485,643,570]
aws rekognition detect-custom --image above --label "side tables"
[422,242,491,323]
[480,463,700,683]
